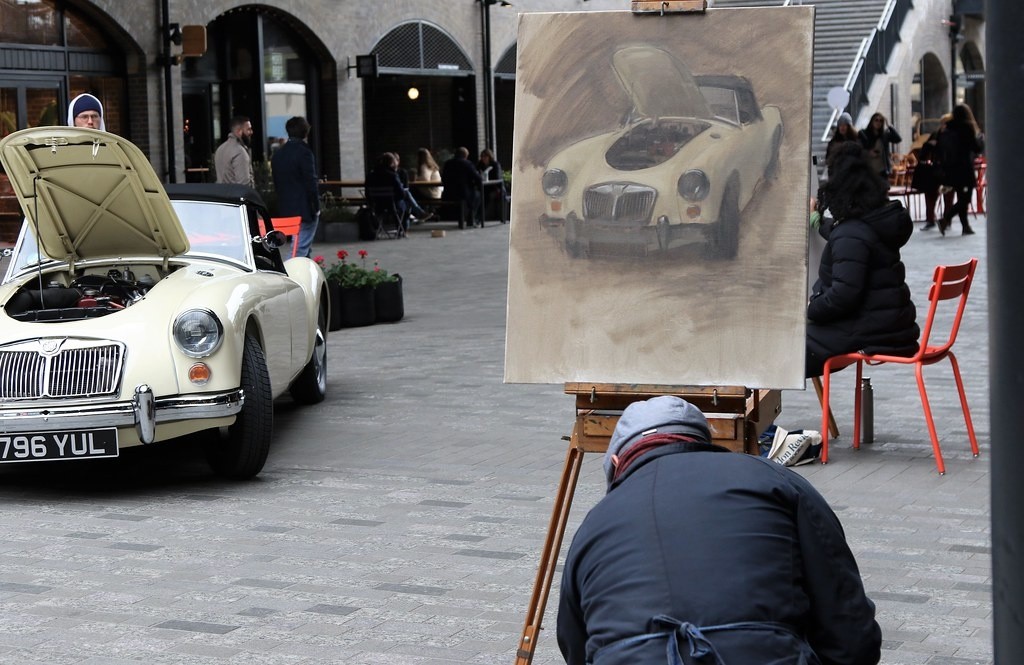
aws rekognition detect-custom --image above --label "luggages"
[356,188,378,241]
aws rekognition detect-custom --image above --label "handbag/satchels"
[758,424,823,467]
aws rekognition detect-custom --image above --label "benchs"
[319,196,474,230]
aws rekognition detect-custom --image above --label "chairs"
[821,259,980,476]
[888,160,986,219]
[889,152,912,186]
[358,186,411,240]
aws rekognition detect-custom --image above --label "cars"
[0,125,331,481]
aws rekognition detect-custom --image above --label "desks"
[318,178,506,228]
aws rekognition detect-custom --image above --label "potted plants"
[320,202,359,241]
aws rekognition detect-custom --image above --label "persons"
[271,116,322,261]
[68,93,106,131]
[214,115,260,189]
[364,145,507,238]
[804,139,921,378]
[813,103,985,236]
[556,395,881,665]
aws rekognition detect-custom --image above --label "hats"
[602,395,713,489]
[73,95,101,122]
[837,112,853,126]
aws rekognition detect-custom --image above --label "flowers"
[313,249,397,287]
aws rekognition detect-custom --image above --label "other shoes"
[416,211,434,224]
[920,220,935,231]
[404,228,409,237]
[937,218,948,236]
[962,226,975,235]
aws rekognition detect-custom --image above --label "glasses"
[874,118,883,123]
[77,113,99,122]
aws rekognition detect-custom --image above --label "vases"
[330,273,404,331]
